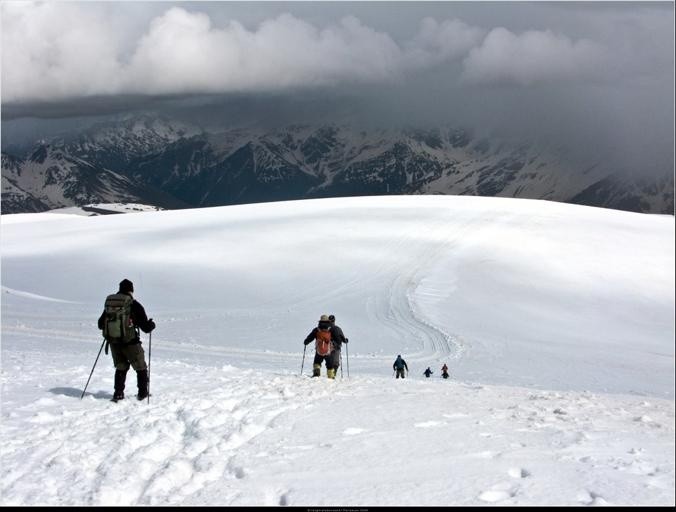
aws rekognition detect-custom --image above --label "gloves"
[147,319,156,330]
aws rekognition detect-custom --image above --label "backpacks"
[315,327,334,356]
[396,359,403,369]
[103,294,136,342]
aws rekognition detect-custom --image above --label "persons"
[422,366,434,379]
[393,354,409,380]
[441,370,449,379]
[441,364,449,372]
[304,314,340,380]
[97,279,156,403]
[329,314,349,377]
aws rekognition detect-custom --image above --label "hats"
[119,279,134,293]
[320,314,336,321]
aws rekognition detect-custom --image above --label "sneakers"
[311,369,337,379]
[112,391,124,399]
[137,390,149,401]
